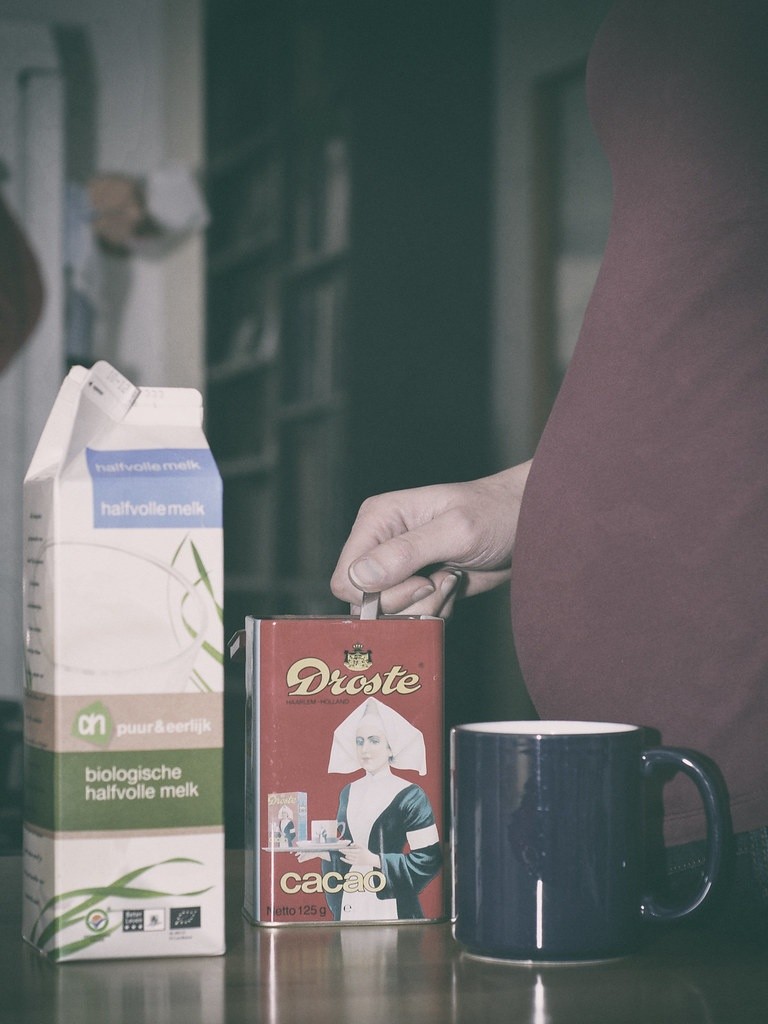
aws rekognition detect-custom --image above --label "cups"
[449,719,736,967]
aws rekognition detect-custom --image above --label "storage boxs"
[227,615,447,927]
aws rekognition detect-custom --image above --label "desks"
[0,851,768,1024]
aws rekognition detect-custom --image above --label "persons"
[278,805,296,847]
[289,695,442,922]
[328,0,767,1022]
